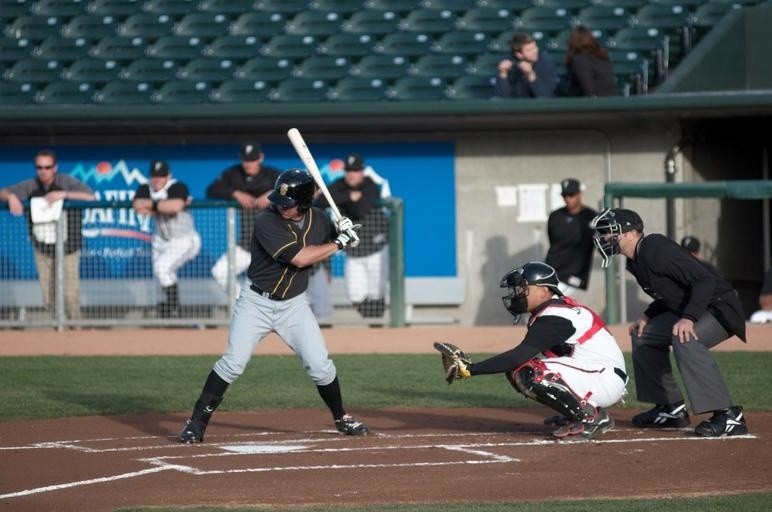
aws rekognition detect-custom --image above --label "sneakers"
[179,419,206,444]
[335,412,369,435]
[547,417,585,438]
[694,405,747,436]
[572,407,615,441]
[633,403,691,428]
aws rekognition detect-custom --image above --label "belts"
[250,283,286,301]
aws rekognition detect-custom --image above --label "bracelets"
[152,199,159,213]
[65,190,70,200]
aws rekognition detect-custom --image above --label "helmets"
[590,207,643,268]
[500,261,564,324]
[267,168,315,213]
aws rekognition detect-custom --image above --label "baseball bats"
[287,127,360,248]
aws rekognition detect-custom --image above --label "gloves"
[337,216,362,232]
[334,228,360,249]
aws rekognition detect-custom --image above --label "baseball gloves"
[433,341,472,384]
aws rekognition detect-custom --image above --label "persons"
[542,178,604,298]
[313,154,389,321]
[747,276,771,323]
[565,26,624,96]
[495,31,567,97]
[430,261,629,441]
[1,149,97,330]
[679,234,721,277]
[307,180,332,328]
[131,160,203,318]
[178,168,370,445]
[205,140,286,300]
[588,207,750,438]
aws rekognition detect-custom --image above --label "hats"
[151,160,168,177]
[345,155,363,169]
[239,140,261,161]
[561,179,579,194]
[681,236,700,252]
